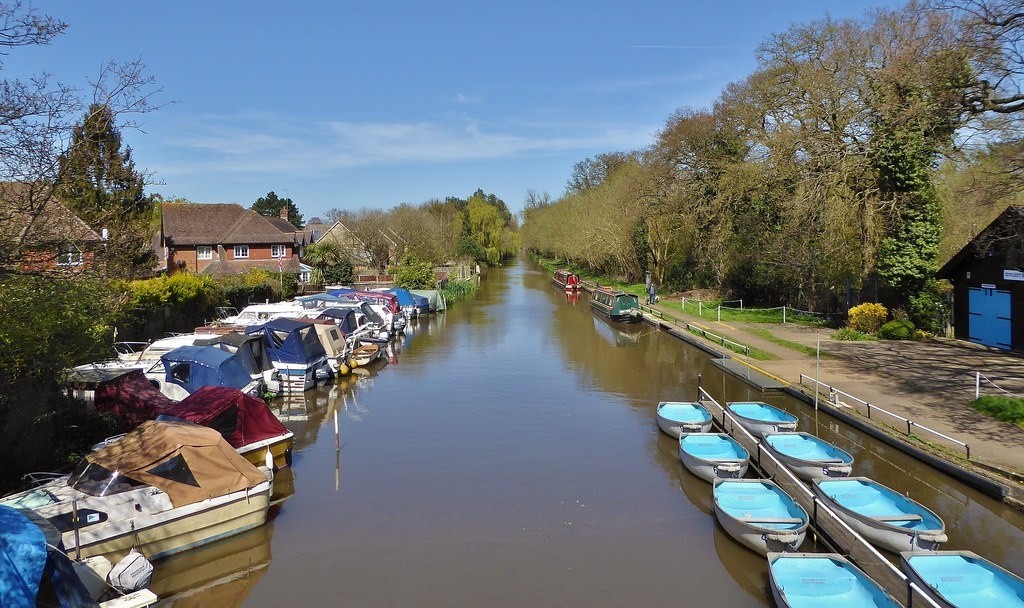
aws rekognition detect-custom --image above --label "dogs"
[655,296,659,303]
[644,296,650,305]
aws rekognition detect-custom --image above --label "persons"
[648,283,656,305]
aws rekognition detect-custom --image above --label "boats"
[759,552,906,608]
[550,267,584,300]
[901,548,1024,608]
[762,433,857,479]
[817,478,952,550]
[27,503,161,608]
[53,286,444,511]
[724,399,809,431]
[654,399,714,432]
[1,422,273,571]
[704,479,813,548]
[673,433,752,479]
[589,286,643,331]
